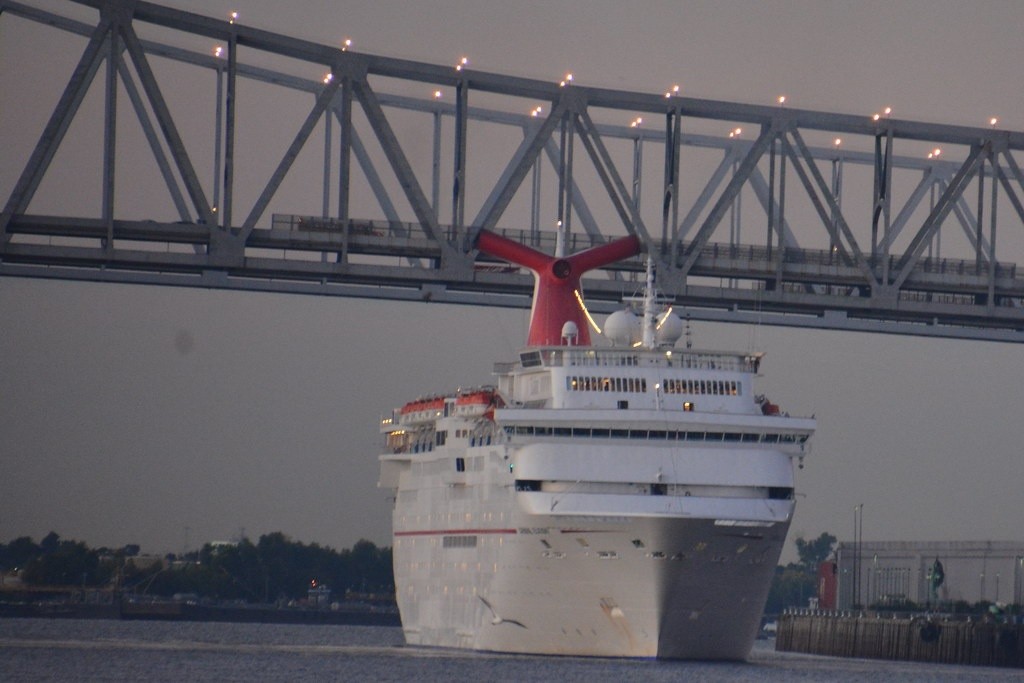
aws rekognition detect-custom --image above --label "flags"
[483,389,505,421]
